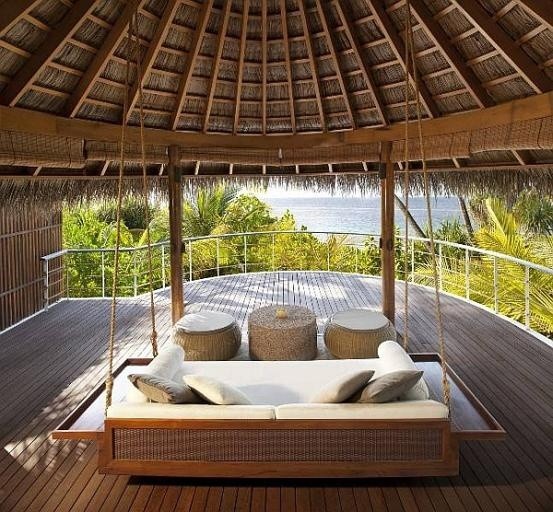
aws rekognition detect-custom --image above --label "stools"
[323,307,398,358]
[169,310,241,361]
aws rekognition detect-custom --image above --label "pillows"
[303,368,425,404]
[125,370,252,407]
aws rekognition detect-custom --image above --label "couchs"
[101,340,460,482]
